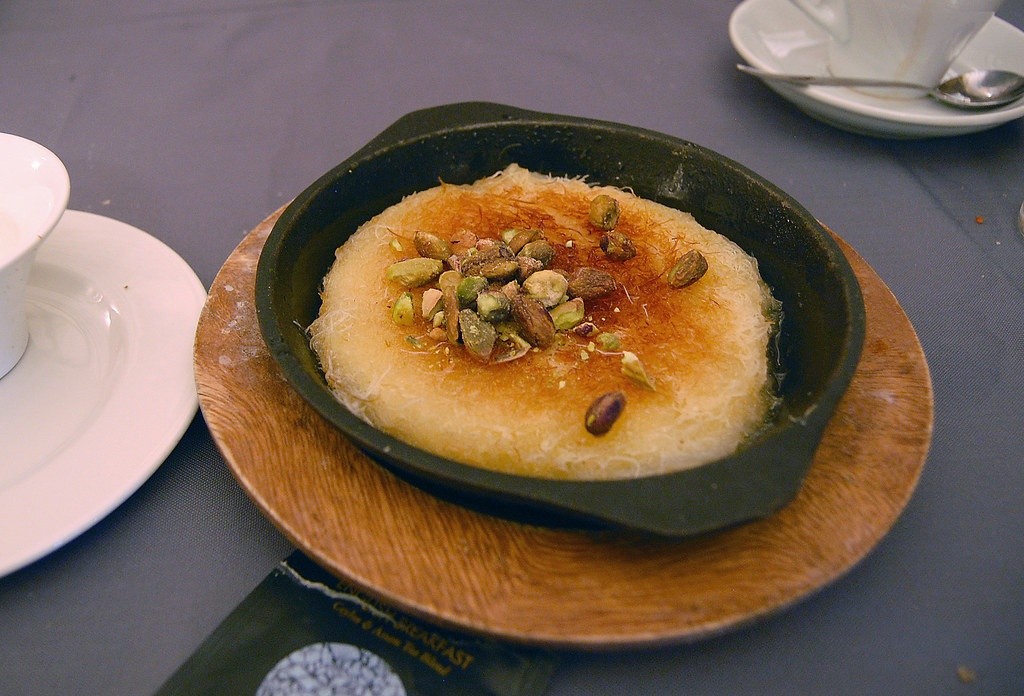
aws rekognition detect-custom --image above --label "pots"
[253,100,867,540]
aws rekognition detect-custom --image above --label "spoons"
[736,64,1024,108]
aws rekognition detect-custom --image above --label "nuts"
[384,194,709,436]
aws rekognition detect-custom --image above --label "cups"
[789,0,1001,100]
[0,132,70,380]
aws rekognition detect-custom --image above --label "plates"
[0,209,208,577]
[728,0,1024,139]
